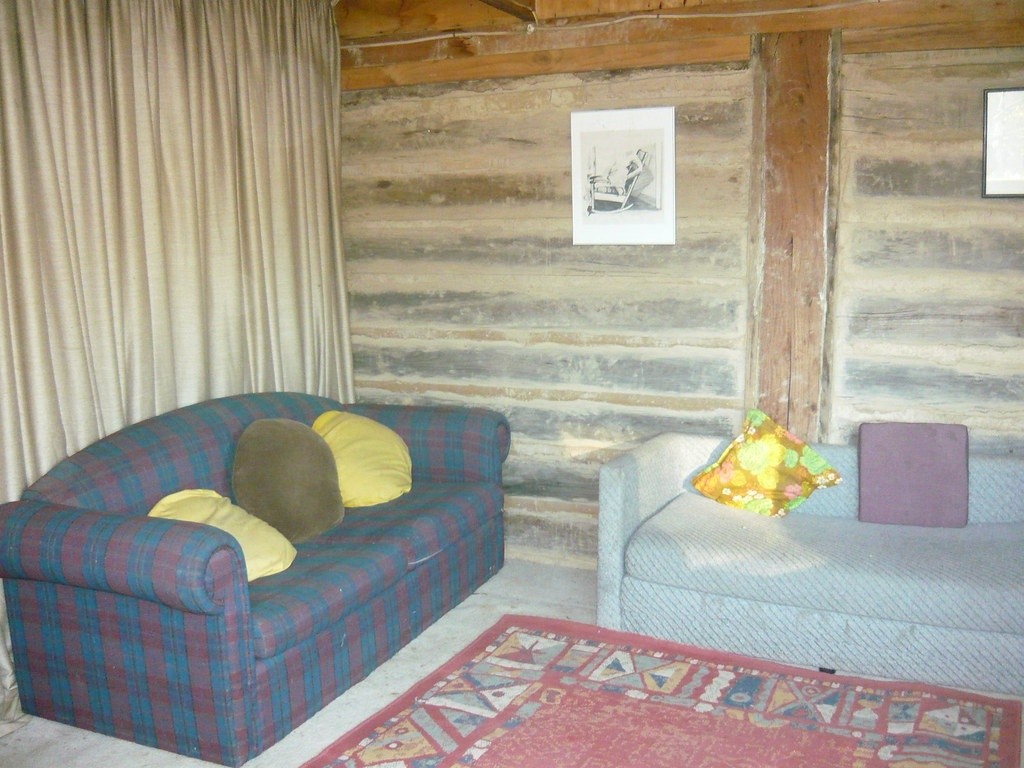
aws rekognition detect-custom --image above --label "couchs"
[598,431,1024,700]
[0,392,510,768]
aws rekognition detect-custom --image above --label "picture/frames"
[572,106,676,246]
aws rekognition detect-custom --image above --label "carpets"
[297,614,1024,768]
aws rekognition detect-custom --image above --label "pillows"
[693,408,843,517]
[233,417,346,544]
[858,423,970,527]
[311,411,413,509]
[147,488,298,583]
